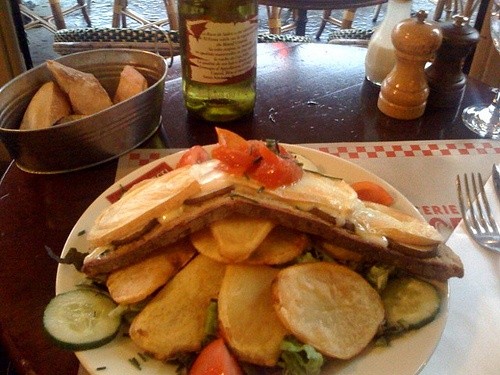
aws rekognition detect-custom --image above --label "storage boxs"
[0,47,171,178]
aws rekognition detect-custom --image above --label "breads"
[80,145,465,280]
[18,59,147,130]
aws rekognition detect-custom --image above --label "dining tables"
[0,40,499,375]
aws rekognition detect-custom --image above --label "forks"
[455,172,500,256]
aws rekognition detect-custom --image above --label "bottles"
[365,0,415,87]
[177,0,257,124]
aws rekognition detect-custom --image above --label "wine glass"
[461,0,500,140]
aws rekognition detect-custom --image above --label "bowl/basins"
[0,48,169,175]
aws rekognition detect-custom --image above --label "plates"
[55,141,450,374]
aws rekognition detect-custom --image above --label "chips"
[104,214,387,368]
